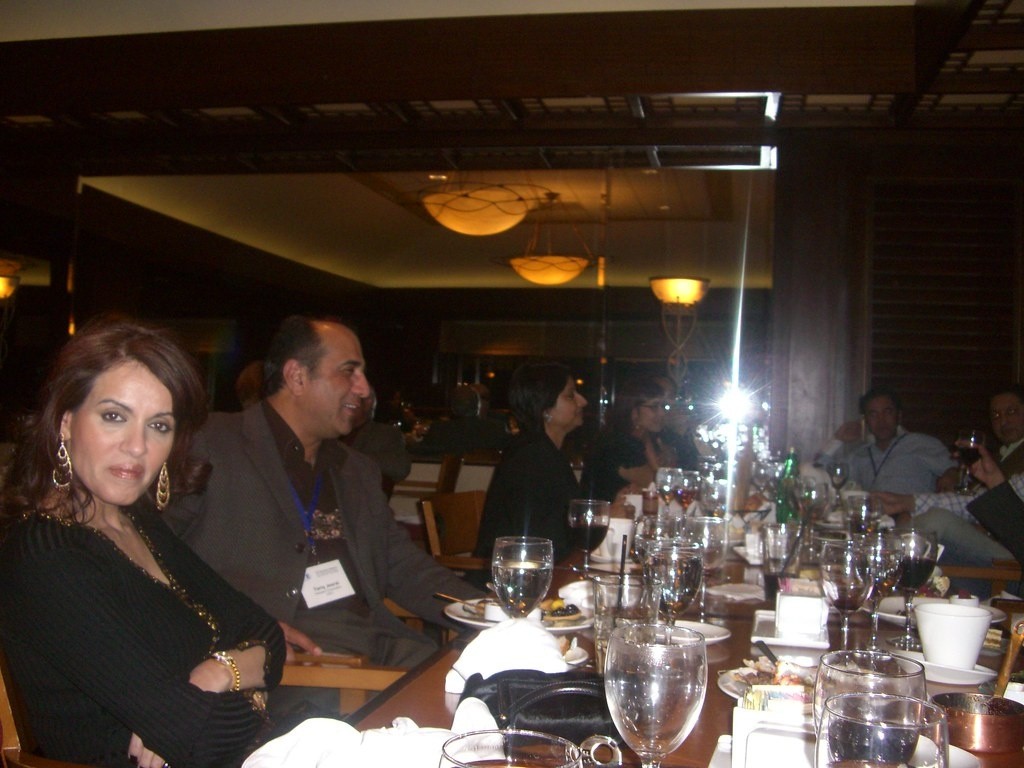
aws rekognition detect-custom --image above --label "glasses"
[639,402,665,411]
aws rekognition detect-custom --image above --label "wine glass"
[656,467,726,516]
[753,459,848,565]
[642,538,703,646]
[604,623,708,768]
[952,429,986,496]
[820,525,939,651]
[567,499,610,579]
[634,515,727,623]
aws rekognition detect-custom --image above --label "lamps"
[490,192,598,286]
[0,257,22,301]
[646,275,710,408]
[418,170,540,237]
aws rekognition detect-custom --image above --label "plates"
[444,596,594,634]
[718,668,813,714]
[587,563,640,574]
[886,651,1000,685]
[860,596,1008,628]
[705,583,767,601]
[621,621,733,647]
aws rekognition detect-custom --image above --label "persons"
[343,357,521,456]
[465,364,643,586]
[811,387,962,530]
[947,434,1023,566]
[157,312,498,715]
[582,359,718,499]
[934,386,1024,499]
[0,313,329,768]
[864,470,1024,600]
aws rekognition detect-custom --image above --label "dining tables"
[346,461,1024,768]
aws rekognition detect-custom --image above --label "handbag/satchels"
[456,666,642,748]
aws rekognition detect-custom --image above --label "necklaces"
[864,429,909,476]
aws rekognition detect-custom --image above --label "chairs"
[1,650,413,768]
[388,453,463,555]
[417,489,493,571]
[458,447,504,467]
[977,599,1024,703]
[931,560,1023,601]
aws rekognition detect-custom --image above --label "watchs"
[204,650,241,695]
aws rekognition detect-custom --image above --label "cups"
[915,602,992,670]
[438,729,624,768]
[491,536,553,620]
[761,523,801,600]
[812,650,949,768]
[843,495,884,534]
[593,573,661,676]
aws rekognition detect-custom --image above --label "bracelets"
[245,683,266,714]
